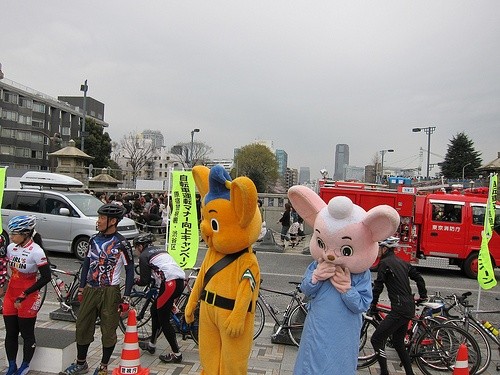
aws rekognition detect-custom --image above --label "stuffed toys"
[287,185,400,375]
[185,165,262,375]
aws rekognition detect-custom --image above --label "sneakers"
[159,352,182,363]
[59,358,89,375]
[93,365,108,375]
[139,341,154,353]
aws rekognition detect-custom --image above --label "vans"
[2,169,142,257]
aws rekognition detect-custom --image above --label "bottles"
[55,278,65,292]
[172,304,179,314]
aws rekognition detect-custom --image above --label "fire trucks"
[316,182,500,281]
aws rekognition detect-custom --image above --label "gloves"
[416,295,430,307]
[366,309,375,316]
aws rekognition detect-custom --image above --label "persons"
[366,236,428,375]
[0,215,52,375]
[432,204,461,223]
[81,189,173,239]
[277,198,306,249]
[195,194,204,242]
[134,233,189,363]
[51,201,66,215]
[59,204,134,375]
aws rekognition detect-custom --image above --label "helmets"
[98,204,126,218]
[133,232,156,246]
[378,236,401,247]
[8,214,36,234]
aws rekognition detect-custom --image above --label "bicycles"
[119,260,202,344]
[357,289,500,375]
[2,254,104,323]
[253,276,309,346]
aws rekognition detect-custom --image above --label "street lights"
[380,149,395,184]
[412,126,436,180]
[189,127,200,170]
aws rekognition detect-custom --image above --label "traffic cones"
[113,309,150,375]
[453,344,469,375]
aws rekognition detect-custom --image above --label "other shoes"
[14,364,30,375]
[5,363,18,375]
[281,245,285,248]
[292,243,295,248]
